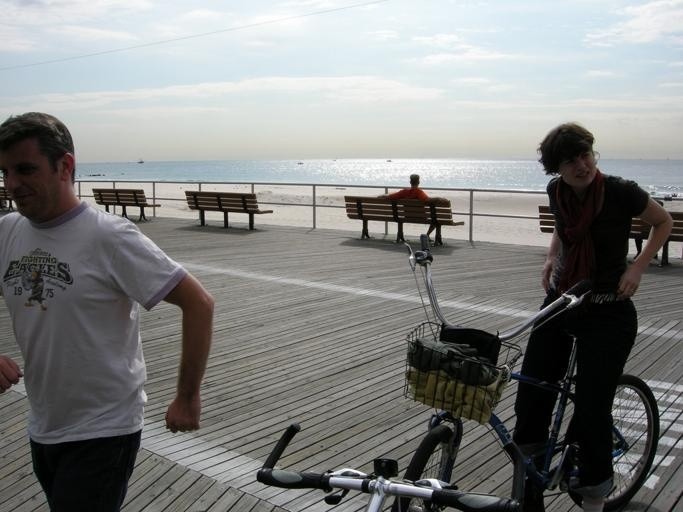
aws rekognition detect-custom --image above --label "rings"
[630,289,635,293]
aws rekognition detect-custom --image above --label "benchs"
[92,188,161,221]
[185,190,273,230]
[538,205,683,267]
[344,196,464,248]
[0,187,15,212]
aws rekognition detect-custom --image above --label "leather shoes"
[569,463,613,489]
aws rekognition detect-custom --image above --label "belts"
[590,293,617,305]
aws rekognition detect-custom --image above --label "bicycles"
[255,421,546,509]
[383,232,662,511]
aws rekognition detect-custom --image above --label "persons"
[0,110,216,511]
[633,200,664,259]
[506,123,667,510]
[373,171,450,243]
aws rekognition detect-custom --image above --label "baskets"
[403,322,522,425]
